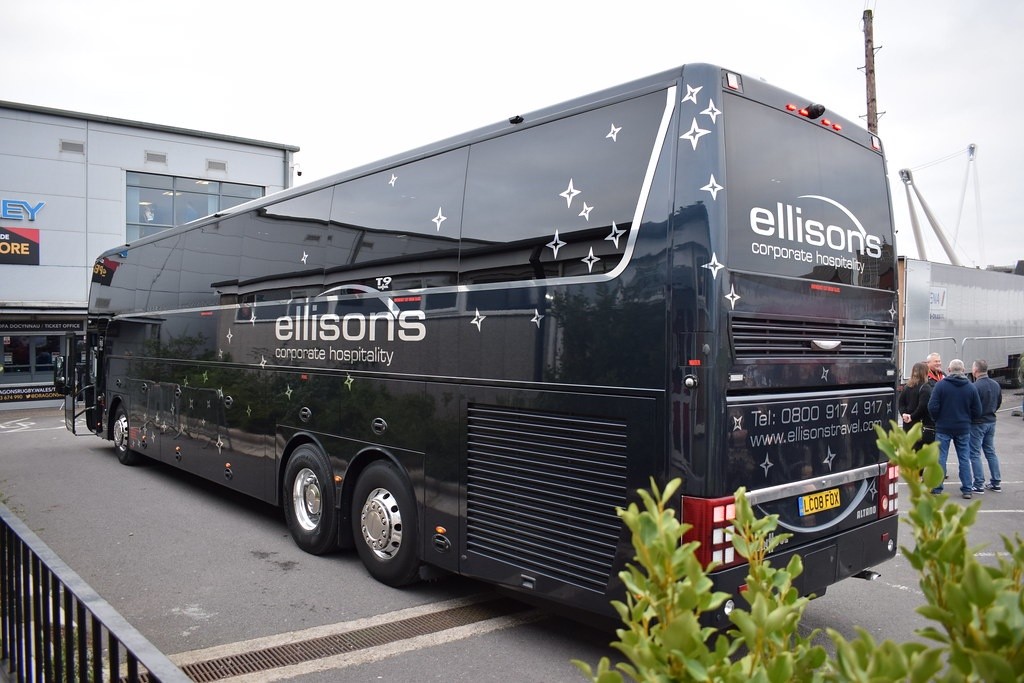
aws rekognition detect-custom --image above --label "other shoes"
[962,492,972,499]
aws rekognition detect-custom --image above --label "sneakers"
[972,479,1002,493]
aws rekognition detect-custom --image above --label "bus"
[53,62,900,653]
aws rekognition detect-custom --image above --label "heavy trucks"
[896,256,1024,403]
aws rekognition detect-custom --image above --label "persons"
[971,360,1003,494]
[926,352,947,389]
[928,359,982,500]
[898,363,932,482]
